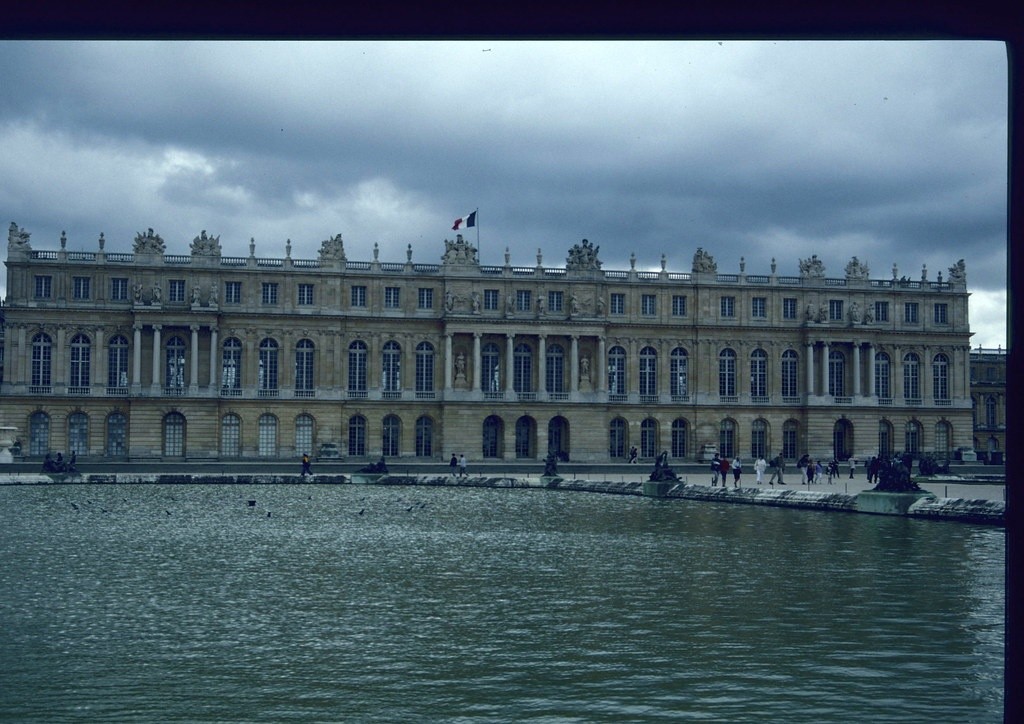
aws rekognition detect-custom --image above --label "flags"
[451,211,477,230]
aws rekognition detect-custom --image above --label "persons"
[459,454,469,477]
[720,455,730,487]
[711,453,722,487]
[455,352,466,377]
[440,234,609,320]
[450,453,458,477]
[753,455,767,484]
[351,455,388,473]
[11,437,76,466]
[579,354,590,374]
[769,447,916,487]
[301,453,313,476]
[690,246,966,325]
[627,446,639,464]
[542,449,559,476]
[650,450,683,481]
[732,456,742,488]
[7,216,346,329]
